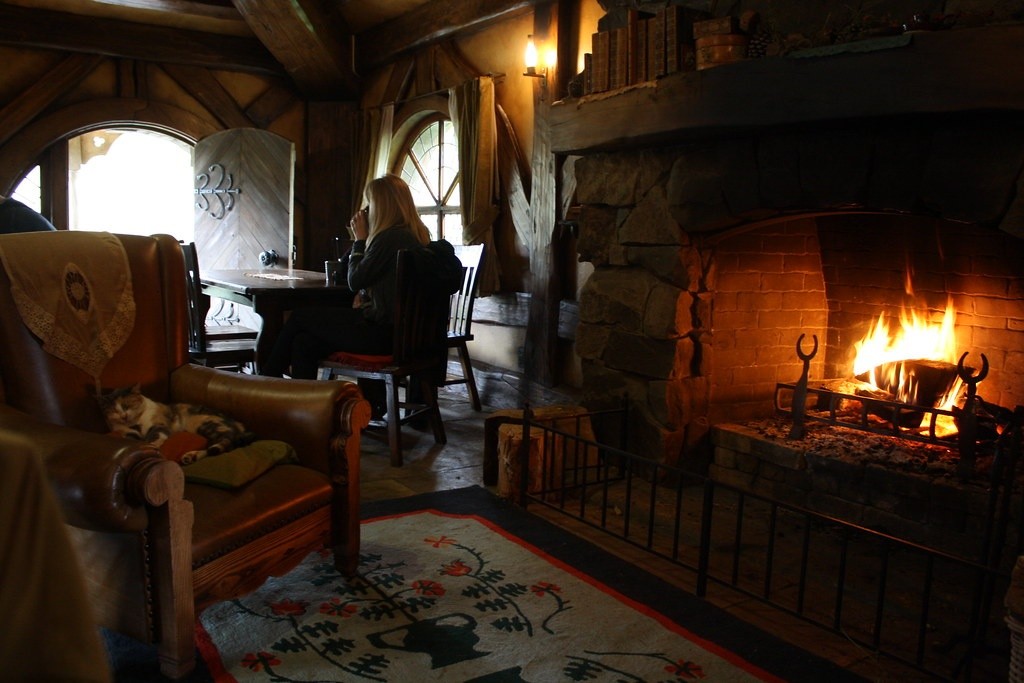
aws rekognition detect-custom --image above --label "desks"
[198,263,364,380]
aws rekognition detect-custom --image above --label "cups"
[325,260,340,288]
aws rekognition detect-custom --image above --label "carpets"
[195,481,873,682]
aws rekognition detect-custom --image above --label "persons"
[262,173,430,381]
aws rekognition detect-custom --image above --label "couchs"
[0,234,369,680]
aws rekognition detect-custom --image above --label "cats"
[91,380,256,464]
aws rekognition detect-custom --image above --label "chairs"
[439,238,485,412]
[181,239,259,369]
[315,247,448,466]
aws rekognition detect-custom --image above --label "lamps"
[522,34,547,79]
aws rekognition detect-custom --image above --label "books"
[584,4,711,95]
